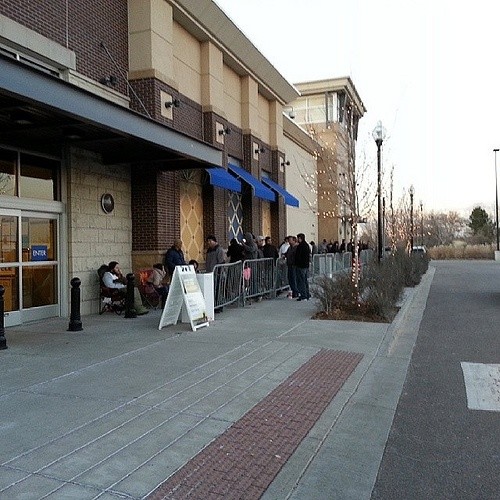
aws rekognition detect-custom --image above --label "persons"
[309,241,317,258]
[205,234,225,313]
[278,232,312,301]
[226,232,280,304]
[147,263,168,309]
[319,238,347,254]
[102,261,150,316]
[165,239,187,280]
[189,260,200,273]
[347,239,362,258]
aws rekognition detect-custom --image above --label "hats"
[256,236,265,240]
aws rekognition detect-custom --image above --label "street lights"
[493,149,500,251]
[407,184,416,252]
[418,199,424,246]
[371,119,391,264]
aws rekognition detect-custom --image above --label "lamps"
[99,75,116,86]
[255,145,266,154]
[165,99,180,108]
[281,161,290,167]
[219,127,231,135]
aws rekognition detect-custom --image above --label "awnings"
[227,164,275,202]
[261,176,299,207]
[206,168,242,193]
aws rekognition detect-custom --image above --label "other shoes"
[297,298,308,301]
[297,294,299,296]
[215,308,223,313]
[287,294,297,297]
[137,307,149,314]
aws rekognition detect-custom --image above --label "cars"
[412,245,426,255]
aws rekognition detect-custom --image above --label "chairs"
[98,264,126,316]
[136,267,170,311]
